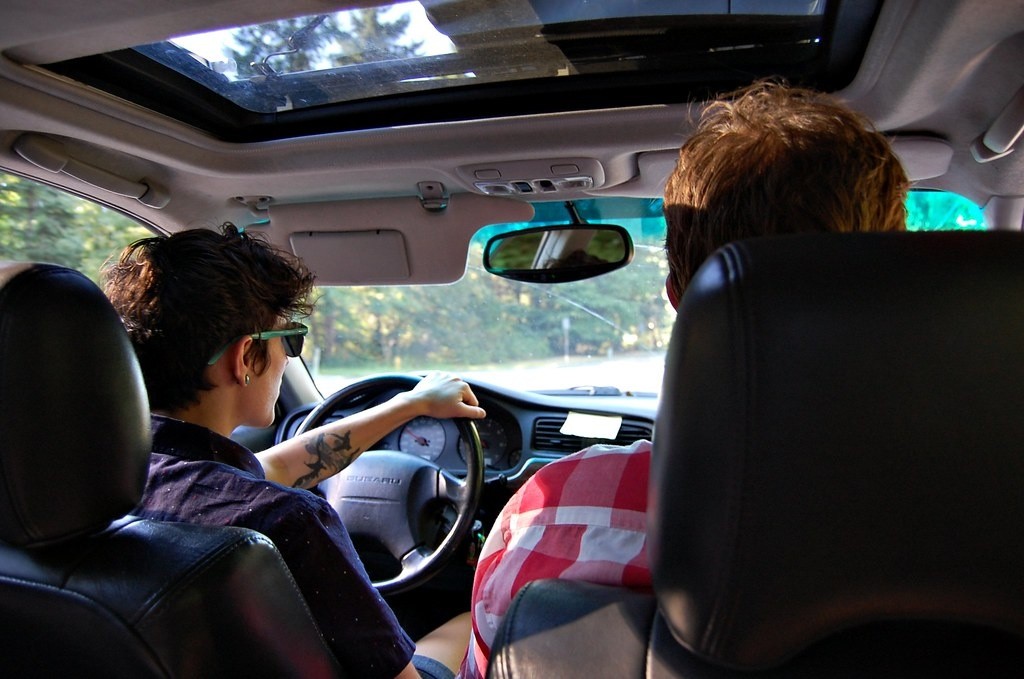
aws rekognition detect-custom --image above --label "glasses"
[208,322,308,366]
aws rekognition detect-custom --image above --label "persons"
[104,220,486,679]
[458,83,907,679]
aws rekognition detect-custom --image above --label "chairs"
[1,264,344,679]
[485,230,1024,679]
